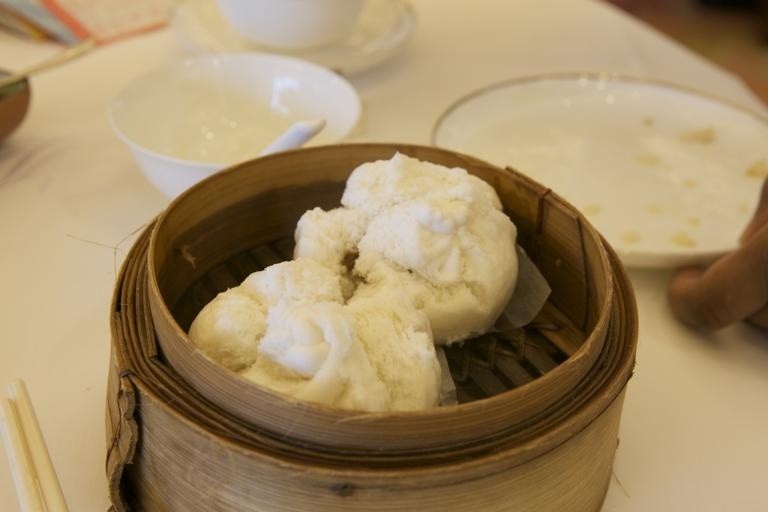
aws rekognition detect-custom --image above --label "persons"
[665,176,766,334]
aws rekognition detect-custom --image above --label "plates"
[430,77,768,273]
[321,2,419,72]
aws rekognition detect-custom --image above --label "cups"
[0,69,30,144]
[121,54,362,204]
[213,0,371,53]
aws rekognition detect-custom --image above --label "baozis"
[187,259,441,414]
[294,152,519,345]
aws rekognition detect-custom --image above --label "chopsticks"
[0,376,71,511]
